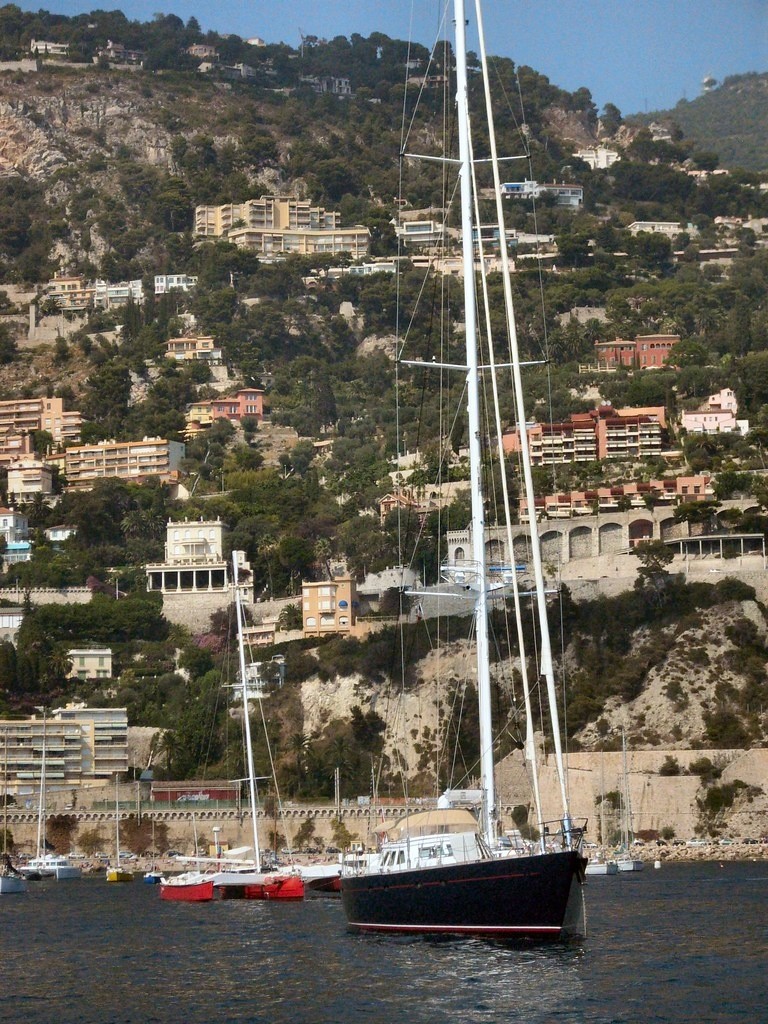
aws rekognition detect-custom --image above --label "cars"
[326,846,341,854]
[281,847,298,855]
[119,850,135,858]
[95,852,111,858]
[634,837,758,847]
[167,851,183,857]
[582,840,598,849]
[303,848,322,854]
[69,852,85,860]
[19,853,34,860]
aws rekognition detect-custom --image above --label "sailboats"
[0,727,26,894]
[17,705,72,878]
[340,0,589,952]
[616,728,643,871]
[585,748,618,875]
[105,551,342,901]
